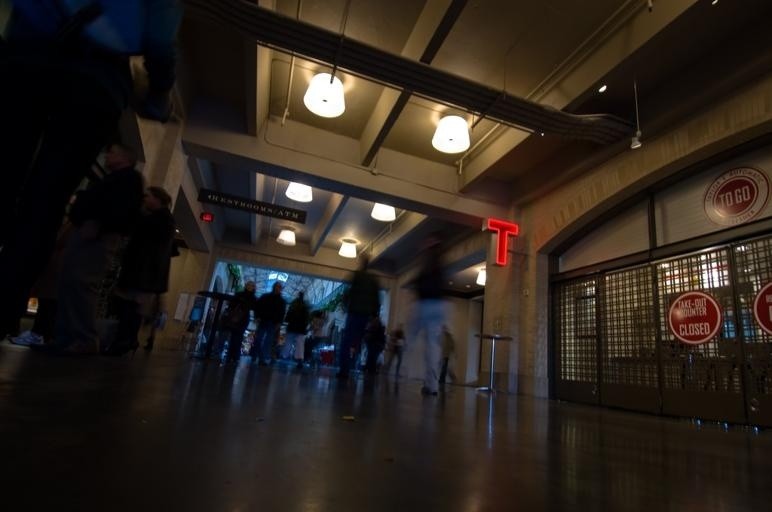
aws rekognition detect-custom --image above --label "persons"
[336,257,380,381]
[214,281,256,362]
[254,282,287,367]
[435,323,456,384]
[380,327,406,379]
[282,292,309,368]
[10,146,174,358]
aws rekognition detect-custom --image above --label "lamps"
[338,237,360,258]
[303,0,351,118]
[629,74,643,149]
[476,267,487,285]
[370,202,397,222]
[432,115,471,155]
[276,225,297,247]
[284,182,312,203]
